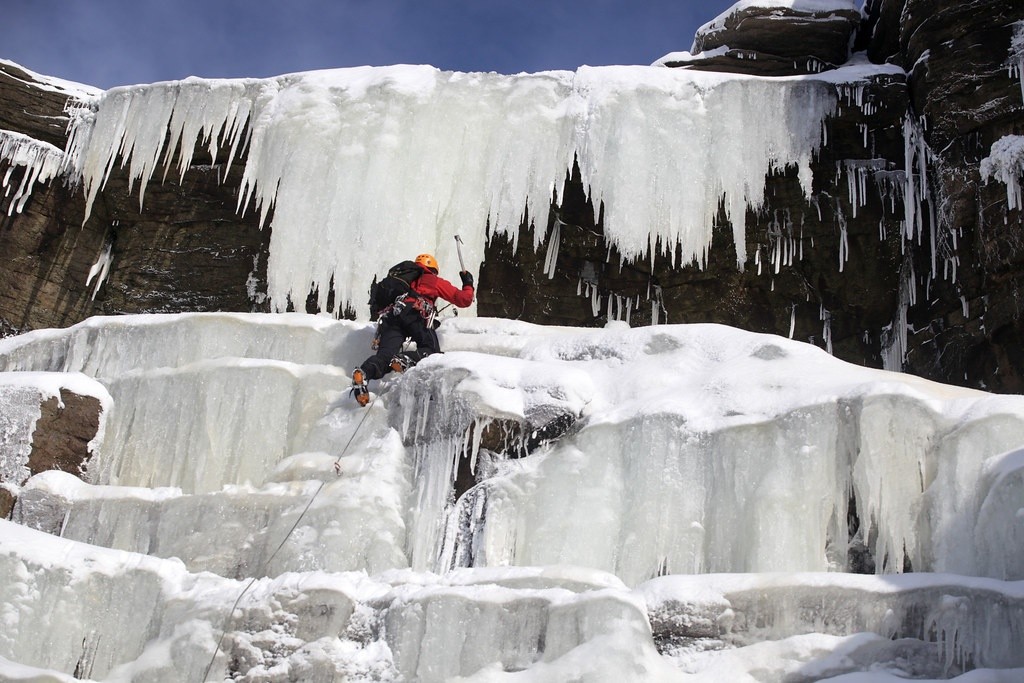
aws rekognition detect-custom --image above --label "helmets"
[415,254,438,275]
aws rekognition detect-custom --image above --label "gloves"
[458,271,475,290]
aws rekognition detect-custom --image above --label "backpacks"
[370,261,436,323]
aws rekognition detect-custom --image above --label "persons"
[351,253,475,407]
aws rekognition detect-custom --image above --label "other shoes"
[349,367,370,406]
[390,354,416,373]
